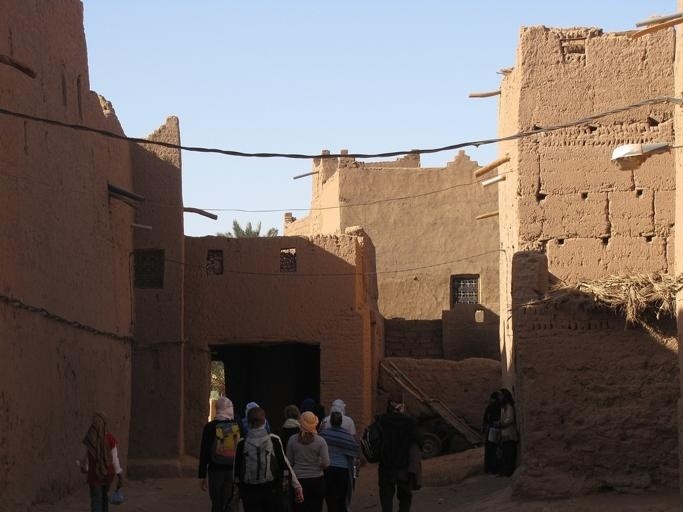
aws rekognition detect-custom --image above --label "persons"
[490,388,516,477]
[195,396,359,512]
[482,390,501,474]
[79,410,123,512]
[369,395,424,511]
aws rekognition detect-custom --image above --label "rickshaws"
[379,357,484,460]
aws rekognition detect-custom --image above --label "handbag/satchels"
[110,489,125,503]
[361,423,384,463]
[224,496,243,512]
[487,428,501,444]
